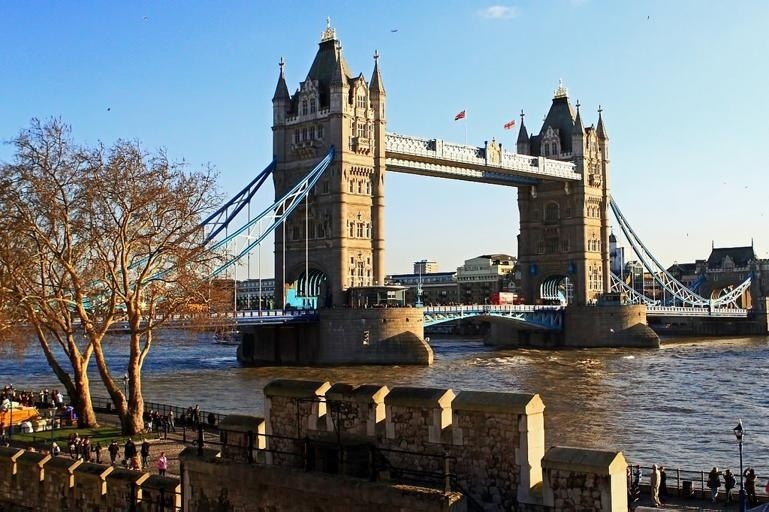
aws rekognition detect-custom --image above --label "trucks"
[489,291,526,305]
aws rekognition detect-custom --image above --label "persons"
[723,469,737,503]
[743,466,759,504]
[626,468,632,490]
[659,465,667,499]
[422,299,561,306]
[707,467,722,504]
[651,463,661,507]
[0,385,201,476]
[634,463,641,483]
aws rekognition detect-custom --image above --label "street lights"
[731,418,750,511]
[47,399,58,455]
[121,373,130,400]
[6,382,17,437]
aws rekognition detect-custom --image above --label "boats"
[211,330,241,345]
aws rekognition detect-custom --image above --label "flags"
[503,120,515,130]
[455,111,465,120]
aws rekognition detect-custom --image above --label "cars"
[536,298,550,305]
